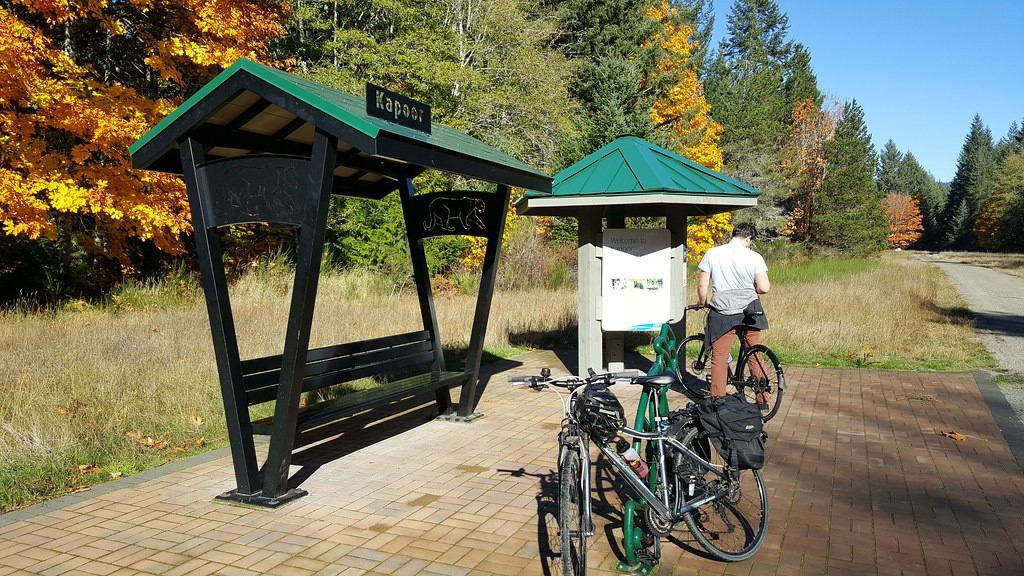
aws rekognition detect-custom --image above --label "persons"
[698,222,772,411]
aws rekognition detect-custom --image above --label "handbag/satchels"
[655,393,768,502]
[579,389,626,440]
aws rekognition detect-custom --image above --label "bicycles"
[675,302,786,422]
[510,366,769,576]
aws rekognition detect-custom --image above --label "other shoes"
[755,403,769,412]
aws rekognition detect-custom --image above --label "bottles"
[615,439,649,478]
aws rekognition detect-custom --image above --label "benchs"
[238,335,478,494]
